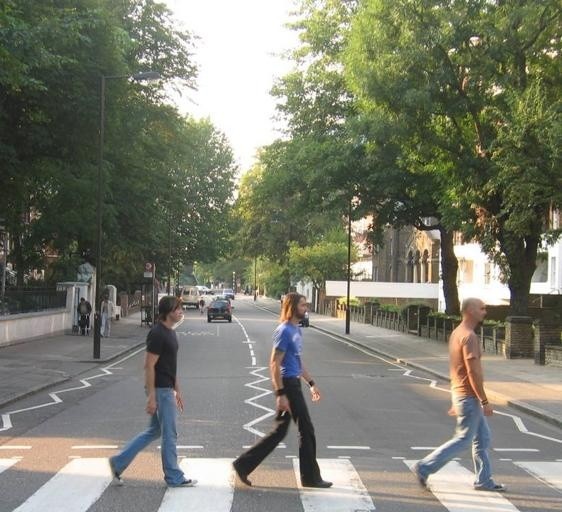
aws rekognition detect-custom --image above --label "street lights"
[93,72,162,364]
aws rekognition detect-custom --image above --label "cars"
[213,295,231,305]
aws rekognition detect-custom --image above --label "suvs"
[181,285,218,309]
[222,288,234,299]
[206,299,234,323]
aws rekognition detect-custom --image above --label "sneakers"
[168,479,197,487]
[232,461,251,486]
[474,483,506,491]
[301,479,332,487]
[109,458,123,485]
[412,462,430,492]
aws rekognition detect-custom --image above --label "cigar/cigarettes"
[280,412,286,416]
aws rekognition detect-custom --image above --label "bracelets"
[480,399,489,406]
[275,388,287,396]
[306,380,315,389]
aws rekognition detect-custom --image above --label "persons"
[100,294,114,337]
[77,297,92,336]
[108,295,198,487]
[232,292,332,487]
[413,298,506,492]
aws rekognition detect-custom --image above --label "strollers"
[72,313,91,336]
